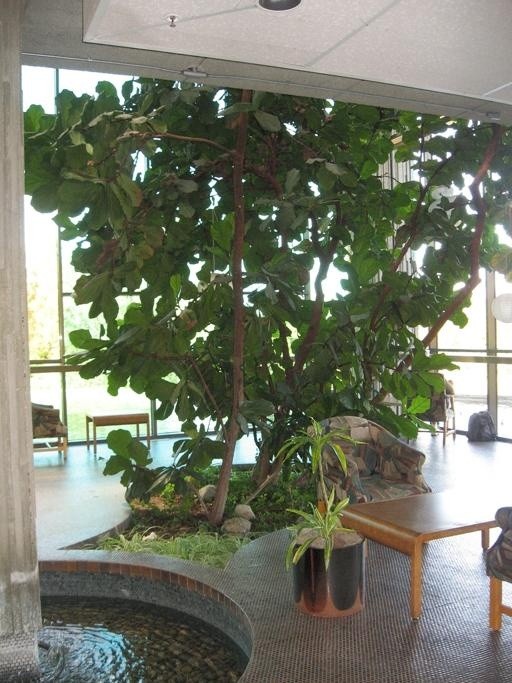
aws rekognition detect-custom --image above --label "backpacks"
[465,409,499,443]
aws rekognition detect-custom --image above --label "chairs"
[32,402,69,459]
[485,509,512,633]
[307,416,432,513]
[406,373,456,446]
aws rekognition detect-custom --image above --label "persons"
[422,354,455,436]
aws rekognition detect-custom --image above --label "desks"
[334,491,504,619]
[85,412,151,454]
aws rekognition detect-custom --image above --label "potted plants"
[271,414,370,618]
